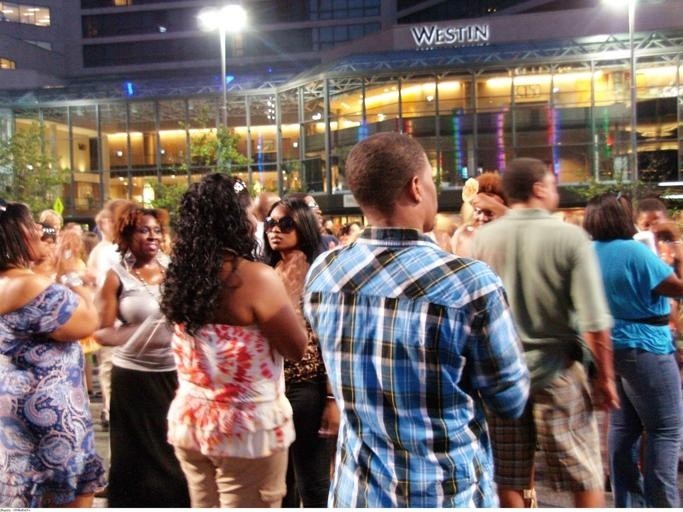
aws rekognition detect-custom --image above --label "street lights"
[605,1,644,188]
[196,3,250,129]
[196,3,247,127]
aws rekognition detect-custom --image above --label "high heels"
[100,409,109,433]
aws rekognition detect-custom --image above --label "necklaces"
[129,257,169,312]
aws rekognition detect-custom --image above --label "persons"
[584,192,683,508]
[54,231,97,398]
[30,210,102,265]
[449,174,507,252]
[91,203,191,508]
[282,187,363,259]
[254,200,341,508]
[302,133,529,508]
[470,159,619,508]
[249,191,282,268]
[166,174,307,509]
[633,194,683,357]
[0,200,110,508]
[88,210,119,429]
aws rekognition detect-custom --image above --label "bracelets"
[324,392,337,401]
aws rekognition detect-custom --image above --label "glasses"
[43,225,56,236]
[308,201,321,210]
[233,175,247,194]
[614,189,628,201]
[264,216,298,236]
